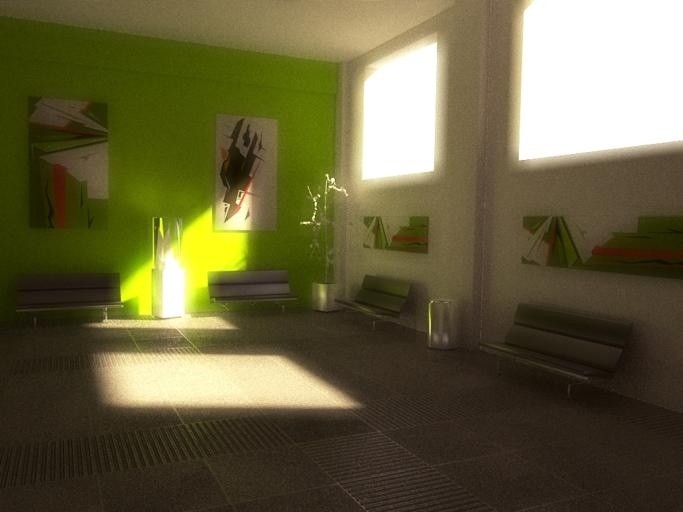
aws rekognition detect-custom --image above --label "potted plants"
[301,169,351,313]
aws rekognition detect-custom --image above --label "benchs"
[12,271,125,327]
[475,298,635,405]
[334,272,412,334]
[207,268,299,317]
